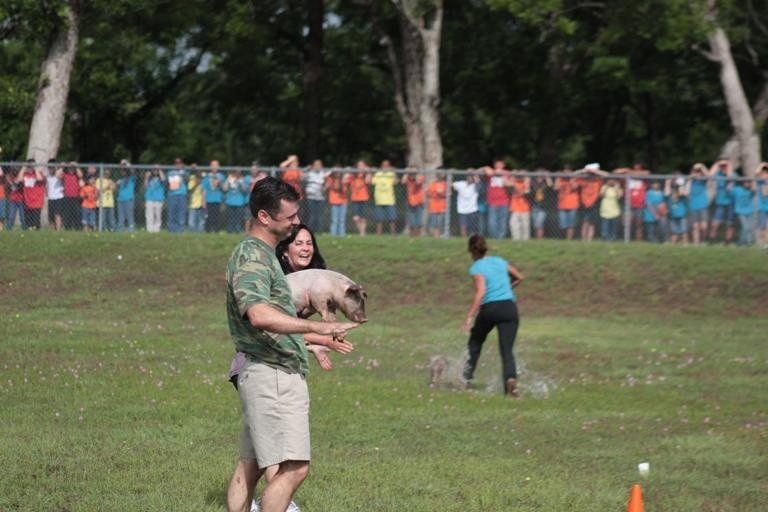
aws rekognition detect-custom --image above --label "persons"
[400,159,768,245]
[222,175,362,512]
[275,221,330,276]
[456,231,527,397]
[2,152,399,237]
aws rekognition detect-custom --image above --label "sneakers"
[449,375,472,390]
[248,496,301,512]
[504,377,521,398]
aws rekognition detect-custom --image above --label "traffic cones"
[628,481,645,512]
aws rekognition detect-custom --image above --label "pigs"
[284,268,369,325]
